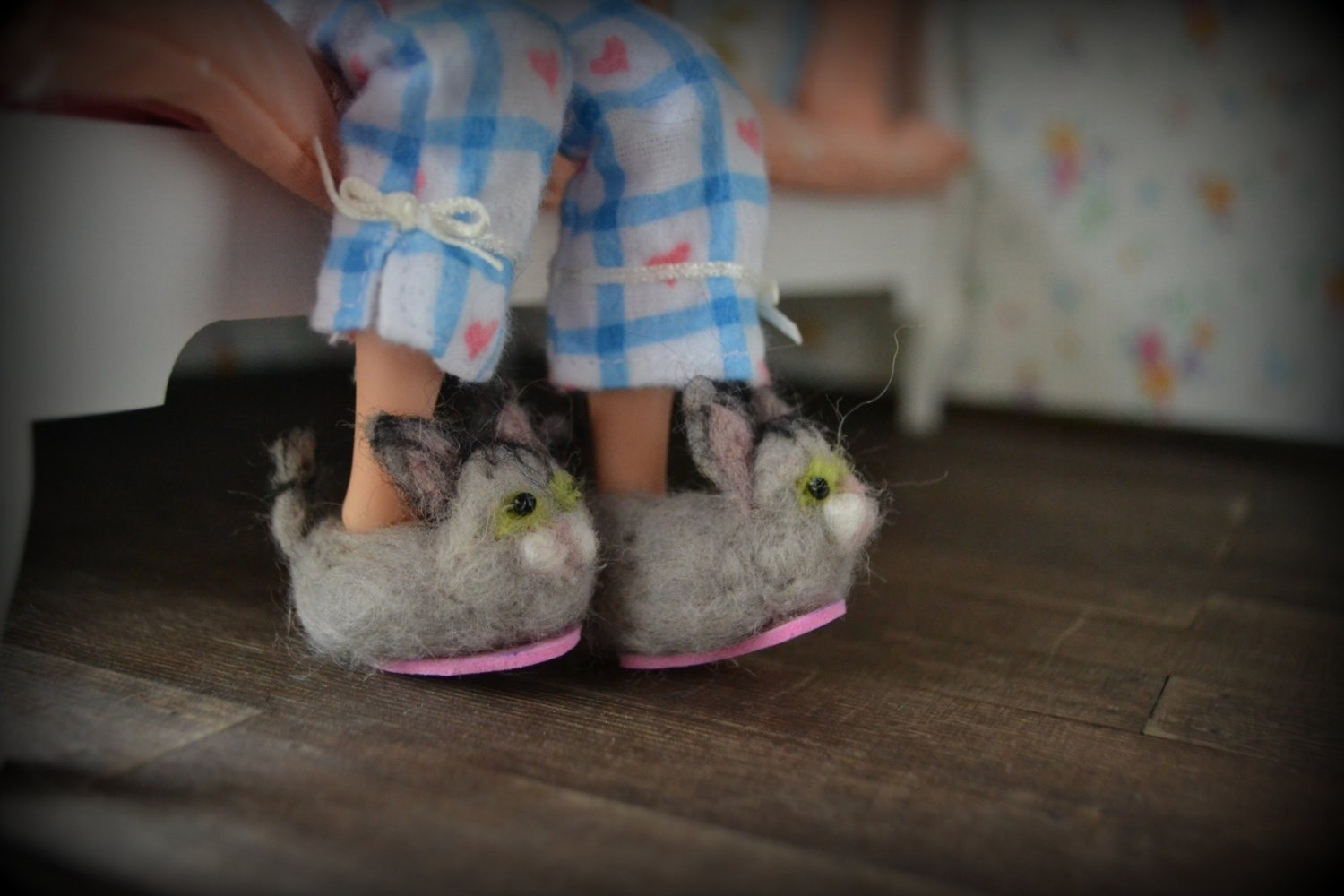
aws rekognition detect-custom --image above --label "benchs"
[0,8,953,620]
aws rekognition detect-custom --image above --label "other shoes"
[267,386,601,677]
[572,374,879,668]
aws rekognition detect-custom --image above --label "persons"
[270,0,884,675]
